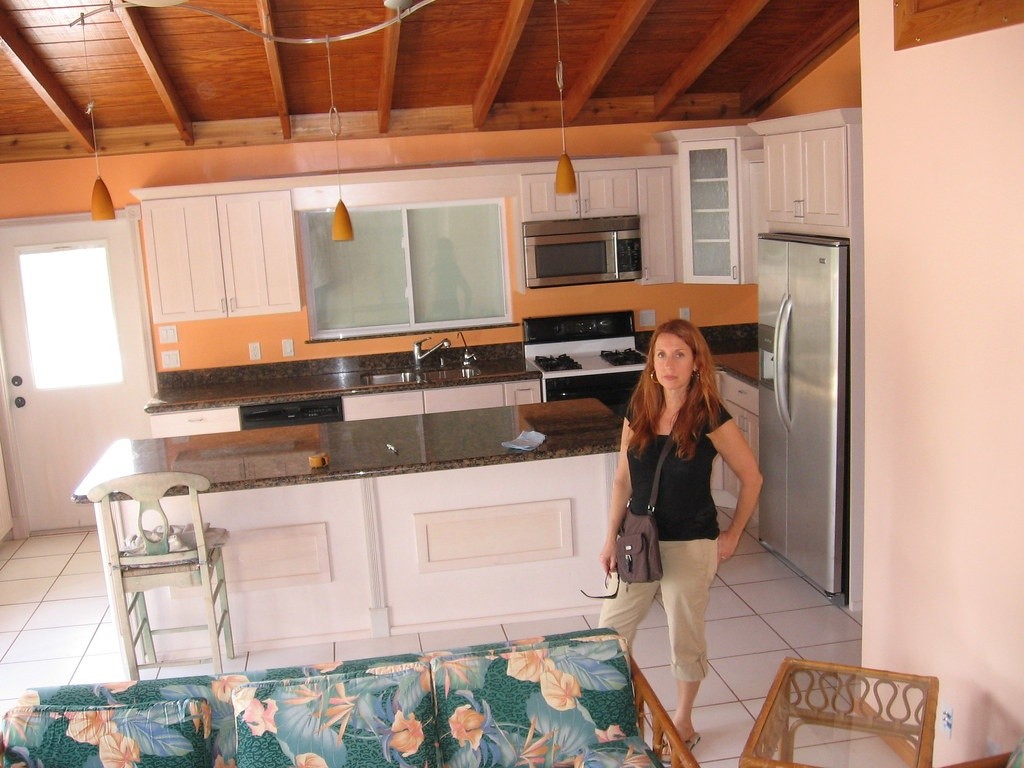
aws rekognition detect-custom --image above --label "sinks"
[361,372,419,386]
[417,365,481,381]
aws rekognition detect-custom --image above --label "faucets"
[413,336,452,367]
[456,331,477,366]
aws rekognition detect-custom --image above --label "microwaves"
[523,214,642,287]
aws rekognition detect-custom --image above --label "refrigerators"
[755,232,865,612]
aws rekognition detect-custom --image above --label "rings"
[722,556,726,559]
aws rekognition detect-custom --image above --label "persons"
[598,319,763,763]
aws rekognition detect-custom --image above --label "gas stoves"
[526,336,650,377]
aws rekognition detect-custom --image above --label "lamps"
[323,34,354,242]
[74,15,116,219]
[548,0,580,195]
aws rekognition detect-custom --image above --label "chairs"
[86,471,235,680]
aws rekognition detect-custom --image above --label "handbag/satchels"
[616,508,663,584]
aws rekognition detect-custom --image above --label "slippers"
[661,732,700,763]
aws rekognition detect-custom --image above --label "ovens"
[540,370,642,418]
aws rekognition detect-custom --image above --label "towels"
[117,521,232,557]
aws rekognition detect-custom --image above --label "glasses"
[581,567,620,599]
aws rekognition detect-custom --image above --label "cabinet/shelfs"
[746,108,862,243]
[652,126,755,283]
[129,179,303,318]
[151,407,241,438]
[721,371,760,512]
[516,155,681,287]
[341,373,541,422]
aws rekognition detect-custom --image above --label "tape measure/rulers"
[307,452,331,469]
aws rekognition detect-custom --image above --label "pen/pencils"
[386,444,399,452]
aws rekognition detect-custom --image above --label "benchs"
[0,628,700,768]
